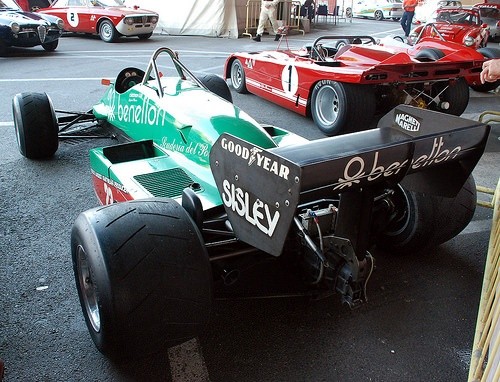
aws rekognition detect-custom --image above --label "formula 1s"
[12,47,490,359]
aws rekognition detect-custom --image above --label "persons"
[401,0,418,36]
[252,0,281,41]
[479,58,500,84]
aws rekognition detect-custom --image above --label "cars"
[407,0,500,51]
[338,0,404,21]
[224,35,488,136]
[0,0,159,51]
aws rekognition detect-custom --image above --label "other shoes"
[274,34,281,41]
[253,36,261,42]
